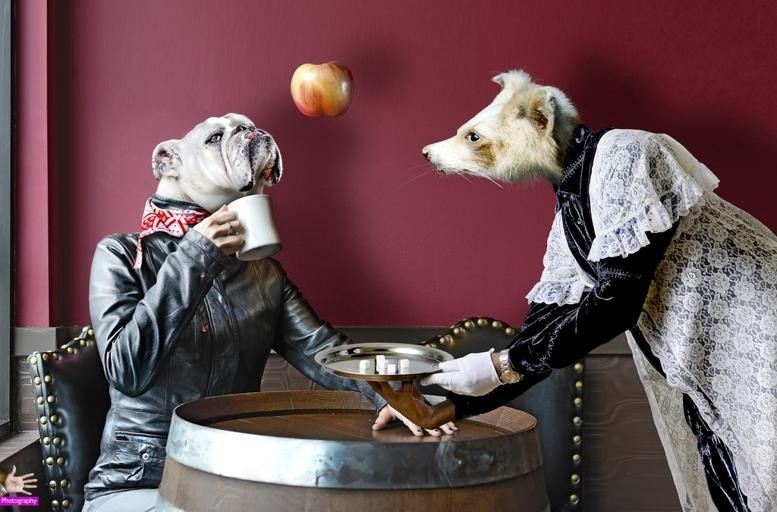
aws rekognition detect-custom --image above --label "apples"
[290,60,352,118]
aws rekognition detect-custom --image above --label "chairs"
[419,315,586,512]
[26,324,111,512]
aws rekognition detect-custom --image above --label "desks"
[153,389,552,511]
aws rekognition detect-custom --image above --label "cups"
[227,195,283,262]
